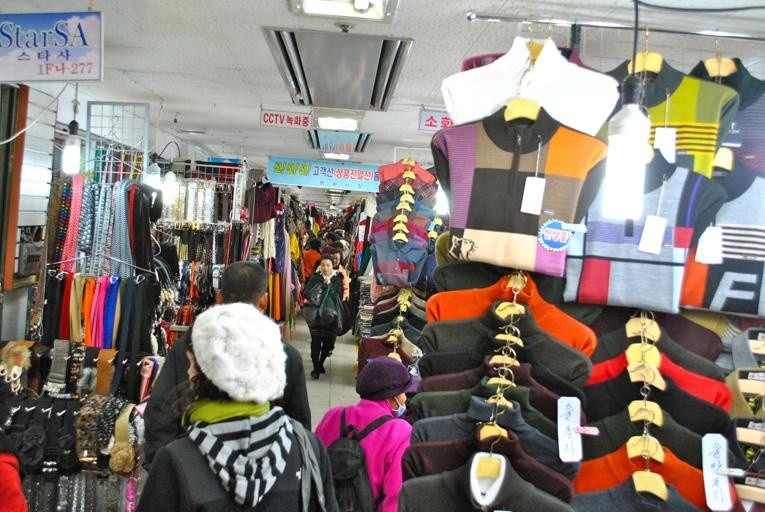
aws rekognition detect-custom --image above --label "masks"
[391,396,407,418]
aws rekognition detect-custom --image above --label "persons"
[314,355,414,512]
[301,218,354,378]
[138,261,313,474]
[134,302,341,511]
[0,380,29,512]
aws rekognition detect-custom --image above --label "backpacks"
[327,408,395,512]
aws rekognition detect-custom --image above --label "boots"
[319,353,326,374]
[311,356,319,379]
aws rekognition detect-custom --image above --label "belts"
[0,340,166,512]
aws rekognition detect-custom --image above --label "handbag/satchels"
[301,284,352,336]
[246,181,277,223]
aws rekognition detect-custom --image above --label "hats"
[356,355,414,399]
[191,302,288,403]
